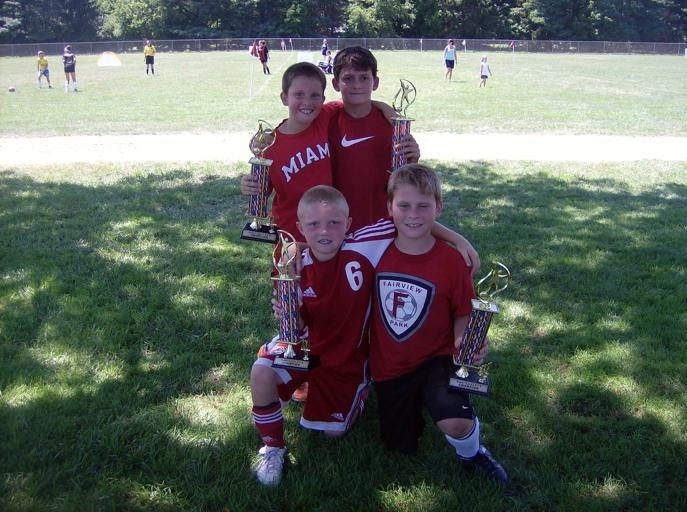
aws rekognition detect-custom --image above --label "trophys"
[383,77,418,190]
[446,260,512,398]
[239,119,281,243]
[269,229,321,373]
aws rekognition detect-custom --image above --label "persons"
[257,39,271,76]
[479,55,493,89]
[326,46,421,238]
[320,39,330,63]
[62,45,78,92]
[143,39,156,75]
[239,60,406,403]
[318,51,333,74]
[248,184,482,487]
[36,50,55,90]
[443,39,457,80]
[275,161,510,491]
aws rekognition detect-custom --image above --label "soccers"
[9,85,16,93]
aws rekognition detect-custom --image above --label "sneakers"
[257,341,274,359]
[462,446,509,486]
[291,382,309,401]
[253,445,286,486]
